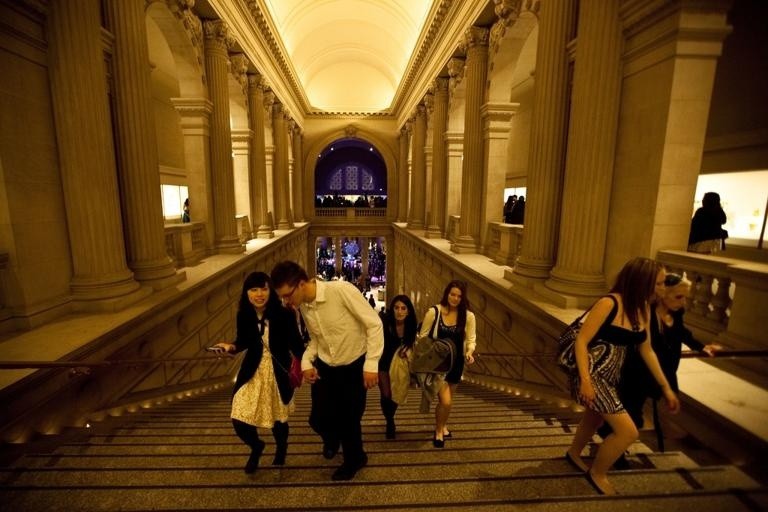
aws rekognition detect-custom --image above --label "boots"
[271,426,289,466]
[380,397,398,440]
[237,427,265,474]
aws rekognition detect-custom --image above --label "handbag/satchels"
[287,357,304,388]
[556,295,618,376]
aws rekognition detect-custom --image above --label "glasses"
[279,278,304,298]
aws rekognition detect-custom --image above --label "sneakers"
[613,453,630,470]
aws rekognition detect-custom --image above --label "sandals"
[565,451,620,495]
[433,429,452,448]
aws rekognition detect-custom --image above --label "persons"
[318,242,386,261]
[688,192,728,253]
[213,261,384,481]
[315,195,387,207]
[378,294,418,439]
[418,280,477,449]
[566,256,723,496]
[182,199,190,222]
[503,195,525,224]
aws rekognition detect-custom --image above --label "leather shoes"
[331,448,368,481]
[323,437,340,460]
